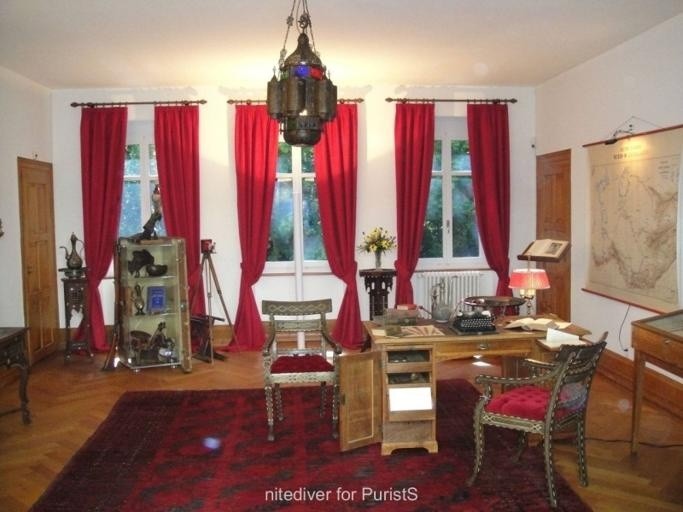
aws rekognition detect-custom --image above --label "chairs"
[259,299,342,442]
[465,340,608,508]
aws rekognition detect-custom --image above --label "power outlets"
[624,346,629,355]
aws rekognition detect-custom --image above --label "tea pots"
[58,231,85,269]
[430,276,452,324]
[128,281,146,315]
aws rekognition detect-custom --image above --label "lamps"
[508,268,550,316]
[266,1,338,148]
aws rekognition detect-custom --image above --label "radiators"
[419,270,482,320]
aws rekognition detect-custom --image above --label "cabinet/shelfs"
[357,267,397,353]
[115,236,192,375]
[61,278,95,367]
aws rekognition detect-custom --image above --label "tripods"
[190,251,243,365]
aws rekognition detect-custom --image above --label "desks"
[629,309,682,455]
[0,327,35,425]
[465,294,527,316]
[330,315,593,460]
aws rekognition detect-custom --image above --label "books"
[500,317,559,332]
[544,326,581,346]
[521,237,568,259]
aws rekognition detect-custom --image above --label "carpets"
[27,378,595,512]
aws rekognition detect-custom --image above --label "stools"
[190,312,229,363]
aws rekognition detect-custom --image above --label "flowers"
[357,226,397,257]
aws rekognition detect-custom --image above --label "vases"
[374,250,381,269]
